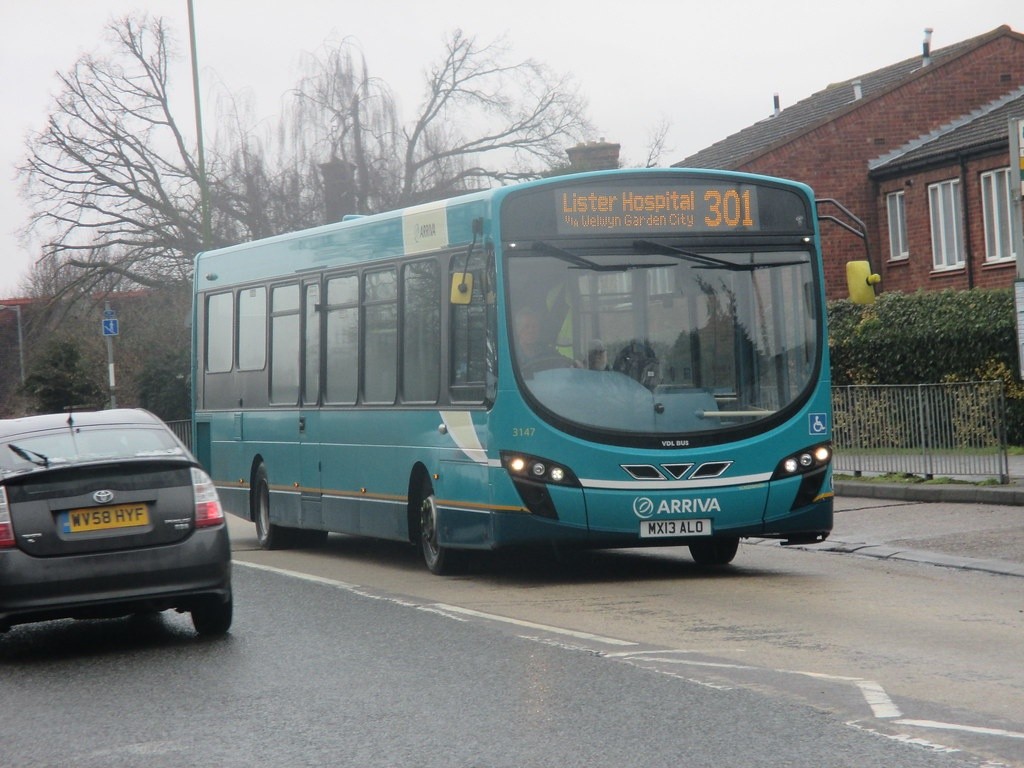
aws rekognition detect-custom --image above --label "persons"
[512,309,572,370]
[583,339,613,372]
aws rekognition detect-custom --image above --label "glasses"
[591,350,605,353]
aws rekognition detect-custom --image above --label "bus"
[190,168,836,578]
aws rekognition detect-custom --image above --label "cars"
[0,407,233,639]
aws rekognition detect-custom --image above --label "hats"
[587,339,606,351]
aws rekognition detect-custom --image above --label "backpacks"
[613,339,660,391]
